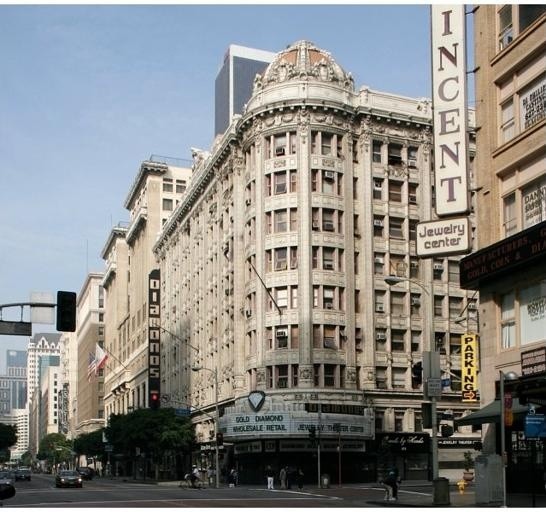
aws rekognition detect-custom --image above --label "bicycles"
[180,476,203,491]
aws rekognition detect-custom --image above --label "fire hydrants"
[456,480,467,494]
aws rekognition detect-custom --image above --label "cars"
[55,471,81,488]
[77,467,92,480]
[0,465,30,501]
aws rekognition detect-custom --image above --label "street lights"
[499,370,518,508]
[190,366,220,489]
[383,276,439,486]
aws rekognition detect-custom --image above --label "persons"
[279,465,287,490]
[188,465,200,489]
[382,475,396,500]
[297,468,304,491]
[266,465,275,489]
[230,467,236,486]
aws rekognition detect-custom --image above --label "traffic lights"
[216,433,223,446]
[308,427,315,442]
[150,390,159,409]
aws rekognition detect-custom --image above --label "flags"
[95,343,108,376]
[87,352,96,383]
